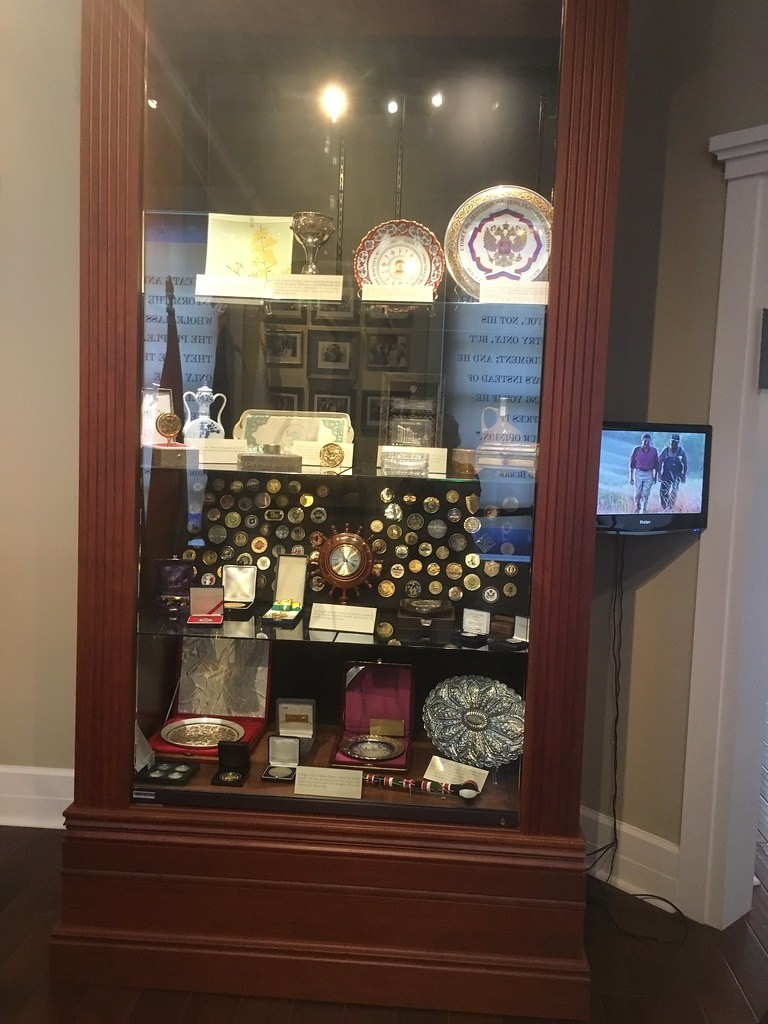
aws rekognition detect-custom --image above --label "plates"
[444,185,553,300]
[339,734,405,761]
[160,718,245,747]
[354,219,444,293]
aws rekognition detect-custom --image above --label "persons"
[629,434,658,514]
[657,435,687,511]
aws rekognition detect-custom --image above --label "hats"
[671,433,679,443]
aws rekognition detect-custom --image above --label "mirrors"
[128,1,563,834]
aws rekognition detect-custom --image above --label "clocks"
[309,522,382,606]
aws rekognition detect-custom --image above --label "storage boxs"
[134,715,200,787]
[330,658,415,774]
[397,606,455,644]
[211,741,251,787]
[153,555,194,616]
[237,452,302,473]
[275,698,317,764]
[221,565,258,615]
[450,607,491,648]
[499,615,530,652]
[187,585,224,627]
[492,615,514,634]
[140,382,199,468]
[147,625,274,764]
[262,554,308,630]
[195,213,344,300]
[261,735,301,783]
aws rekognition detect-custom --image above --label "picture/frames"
[265,282,413,431]
[380,371,447,448]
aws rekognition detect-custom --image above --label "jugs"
[182,374,226,438]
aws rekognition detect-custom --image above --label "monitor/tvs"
[596,421,712,534]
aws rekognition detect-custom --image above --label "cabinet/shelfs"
[47,1,633,1022]
